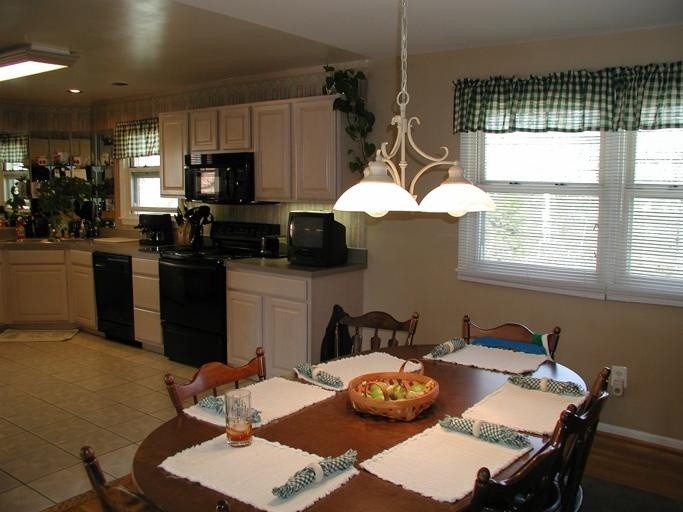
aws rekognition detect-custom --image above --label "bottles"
[15,217,25,240]
[36,156,47,166]
[72,155,81,165]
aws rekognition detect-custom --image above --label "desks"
[131,344,589,509]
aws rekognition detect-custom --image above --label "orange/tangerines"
[370,382,424,417]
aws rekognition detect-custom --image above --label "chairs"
[459,313,561,356]
[561,365,614,511]
[470,410,573,509]
[163,348,269,412]
[328,301,421,354]
[76,439,227,510]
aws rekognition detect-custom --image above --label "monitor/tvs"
[287,211,348,271]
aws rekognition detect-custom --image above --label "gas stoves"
[137,245,264,267]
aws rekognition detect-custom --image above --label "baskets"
[347,357,441,423]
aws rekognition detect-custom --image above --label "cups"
[224,388,252,448]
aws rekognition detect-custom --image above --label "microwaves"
[183,152,282,206]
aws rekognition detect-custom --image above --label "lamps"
[335,0,494,222]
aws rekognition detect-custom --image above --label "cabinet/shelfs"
[252,94,362,205]
[188,104,252,154]
[159,112,188,195]
[66,249,96,331]
[227,269,359,381]
[0,249,70,327]
[128,257,165,358]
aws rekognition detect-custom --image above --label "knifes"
[174,207,183,225]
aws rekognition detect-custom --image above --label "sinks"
[6,239,42,243]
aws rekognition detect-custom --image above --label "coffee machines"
[132,212,173,253]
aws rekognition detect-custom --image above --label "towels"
[506,376,589,396]
[201,394,260,422]
[423,336,466,355]
[439,412,528,448]
[272,446,358,497]
[295,362,343,387]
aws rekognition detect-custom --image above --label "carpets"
[0,323,77,341]
[31,471,683,510]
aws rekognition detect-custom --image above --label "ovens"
[159,261,227,367]
